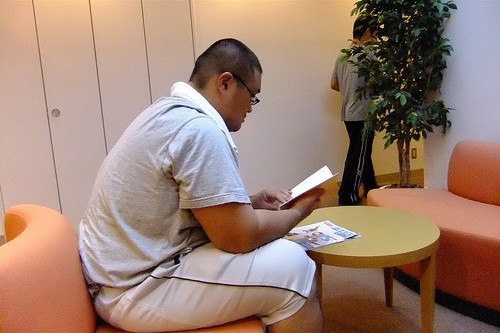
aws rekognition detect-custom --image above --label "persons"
[78,37,326,333]
[331,15,386,206]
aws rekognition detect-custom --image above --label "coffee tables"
[282,206,440,333]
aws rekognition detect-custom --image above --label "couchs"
[0,203,266,333]
[366,140,500,314]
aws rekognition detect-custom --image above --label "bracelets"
[248,196,254,202]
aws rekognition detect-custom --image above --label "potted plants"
[338,0,459,190]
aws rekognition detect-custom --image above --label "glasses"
[233,73,260,106]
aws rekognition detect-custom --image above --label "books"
[279,164,339,210]
[281,219,363,250]
